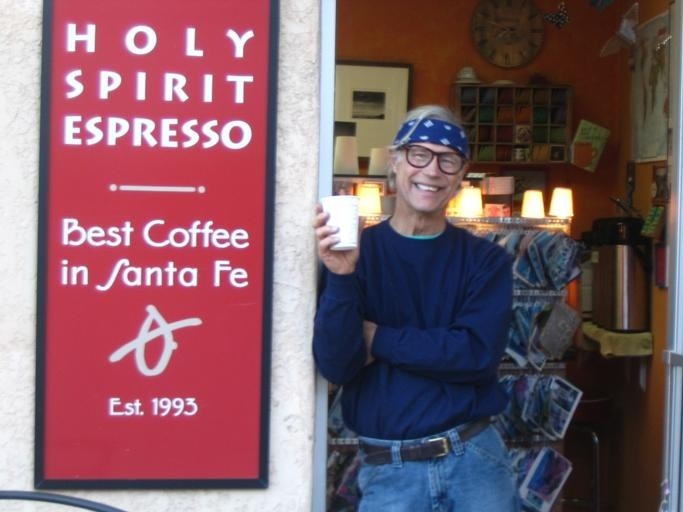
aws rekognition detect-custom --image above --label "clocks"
[467,1,545,70]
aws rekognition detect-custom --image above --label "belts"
[356,413,491,467]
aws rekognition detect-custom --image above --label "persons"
[313,105,524,512]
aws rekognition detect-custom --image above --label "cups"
[571,143,597,165]
[333,136,359,175]
[366,148,388,175]
[497,145,531,162]
[456,66,474,79]
[319,195,360,251]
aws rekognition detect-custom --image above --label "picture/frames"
[335,60,413,178]
[626,10,670,164]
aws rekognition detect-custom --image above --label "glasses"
[398,144,467,176]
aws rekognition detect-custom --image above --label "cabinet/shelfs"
[450,81,574,165]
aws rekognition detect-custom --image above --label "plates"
[478,177,515,196]
